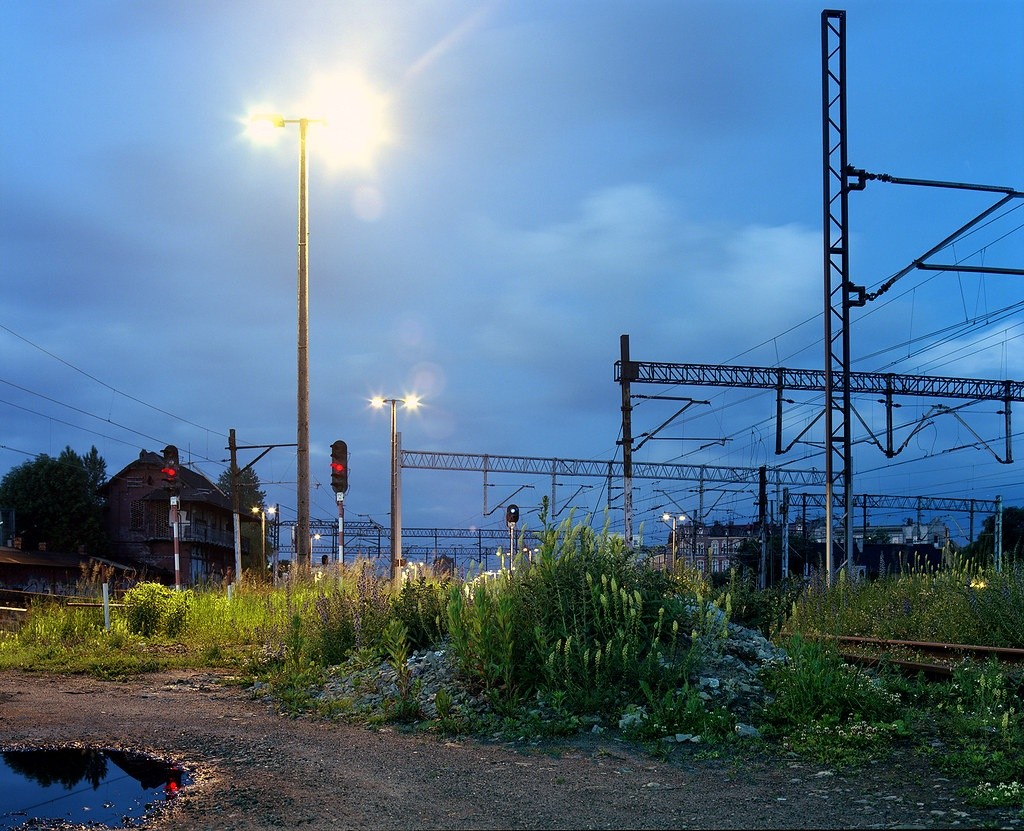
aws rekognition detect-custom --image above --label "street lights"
[310,534,320,568]
[370,391,422,563]
[662,514,686,577]
[252,507,276,582]
[243,86,384,579]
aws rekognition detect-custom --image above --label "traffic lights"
[507,504,519,523]
[163,444,179,483]
[329,439,348,493]
